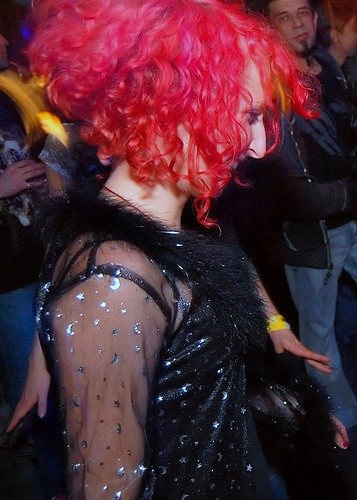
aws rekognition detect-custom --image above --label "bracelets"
[265,315,290,333]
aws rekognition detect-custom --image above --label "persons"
[0,0,357,499]
